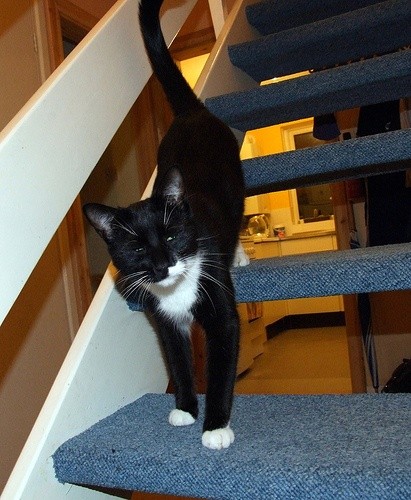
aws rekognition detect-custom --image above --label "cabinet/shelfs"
[241,230,344,358]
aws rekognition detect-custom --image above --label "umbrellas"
[347,200,381,394]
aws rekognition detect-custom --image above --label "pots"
[274,227,286,236]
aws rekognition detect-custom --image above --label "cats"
[81,0,242,450]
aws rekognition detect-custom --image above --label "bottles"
[314,209,318,217]
